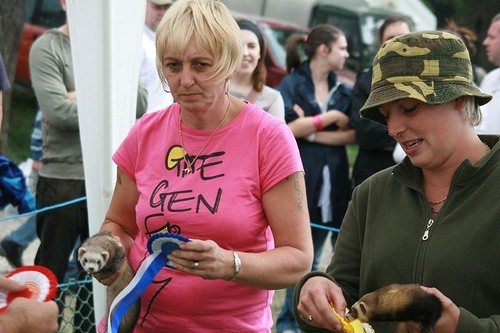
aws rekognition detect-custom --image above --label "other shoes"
[0,240,27,267]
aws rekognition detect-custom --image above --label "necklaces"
[425,193,447,215]
[179,92,231,173]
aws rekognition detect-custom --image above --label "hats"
[359,31,493,126]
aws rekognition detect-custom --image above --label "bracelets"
[224,251,241,281]
[313,114,324,131]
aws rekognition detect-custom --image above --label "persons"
[0,0,94,333]
[292,31,500,333]
[277,24,358,333]
[137,0,285,121]
[350,13,500,199]
[94,0,314,333]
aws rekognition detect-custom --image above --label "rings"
[307,315,313,322]
[192,261,200,271]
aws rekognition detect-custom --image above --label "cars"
[227,0,418,95]
